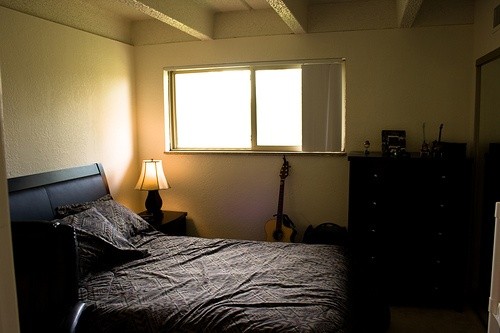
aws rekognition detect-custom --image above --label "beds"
[9,164,354,333]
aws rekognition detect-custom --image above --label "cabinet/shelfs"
[346,153,484,313]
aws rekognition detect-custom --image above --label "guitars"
[264,161,297,243]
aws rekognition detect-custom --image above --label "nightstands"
[136,207,189,236]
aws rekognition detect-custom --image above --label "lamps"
[137,160,170,225]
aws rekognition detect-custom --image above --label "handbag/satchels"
[302,223,346,245]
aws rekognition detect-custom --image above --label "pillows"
[53,194,168,250]
[48,208,150,273]
